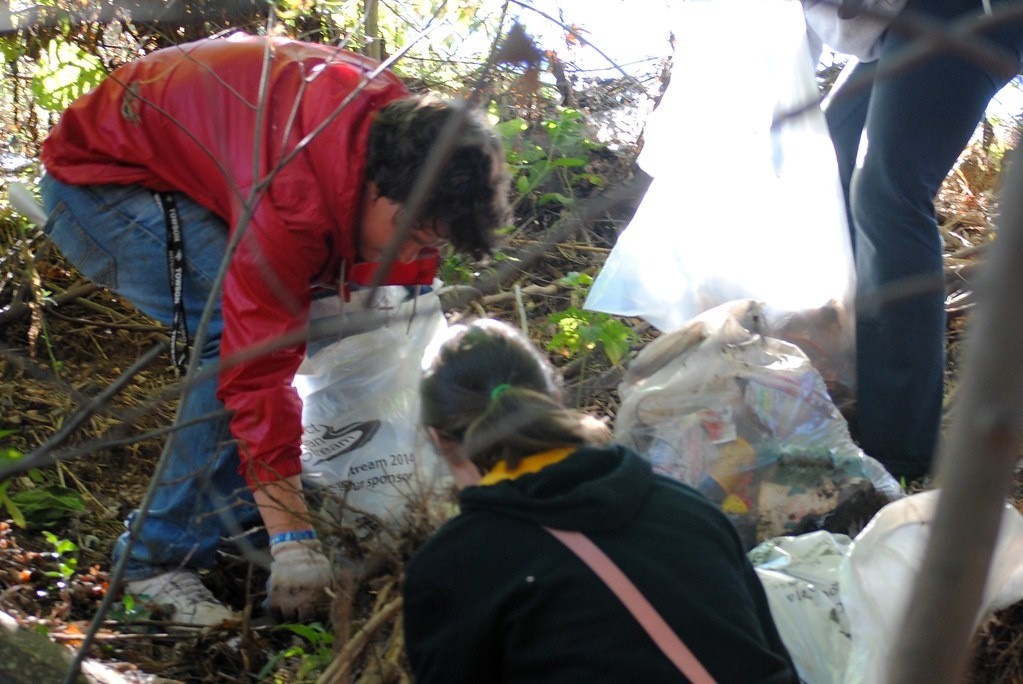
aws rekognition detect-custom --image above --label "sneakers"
[125,570,240,632]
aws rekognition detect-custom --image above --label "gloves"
[261,529,332,619]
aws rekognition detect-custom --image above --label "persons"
[792,0,1023,536]
[41,36,512,639]
[397,318,797,683]
